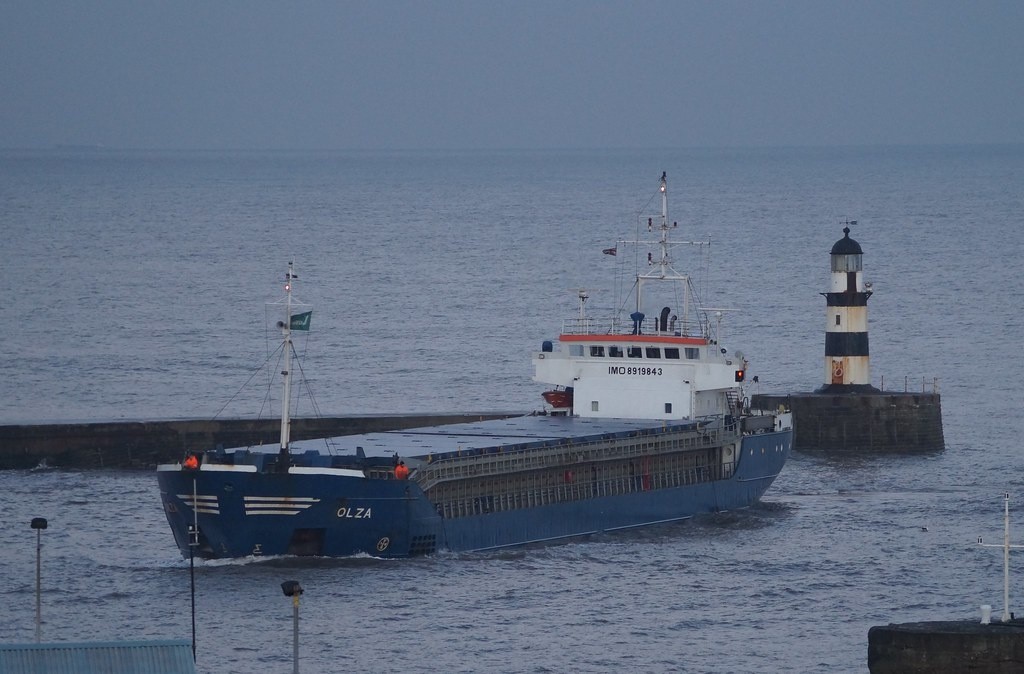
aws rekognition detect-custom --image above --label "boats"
[157,170,794,559]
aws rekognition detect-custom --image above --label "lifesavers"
[539,354,545,359]
[835,368,842,377]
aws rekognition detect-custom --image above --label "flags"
[602,247,616,256]
[284,311,311,331]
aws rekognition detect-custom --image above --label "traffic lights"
[182,449,204,477]
[735,371,744,382]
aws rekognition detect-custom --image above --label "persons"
[184,451,198,469]
[395,461,408,479]
